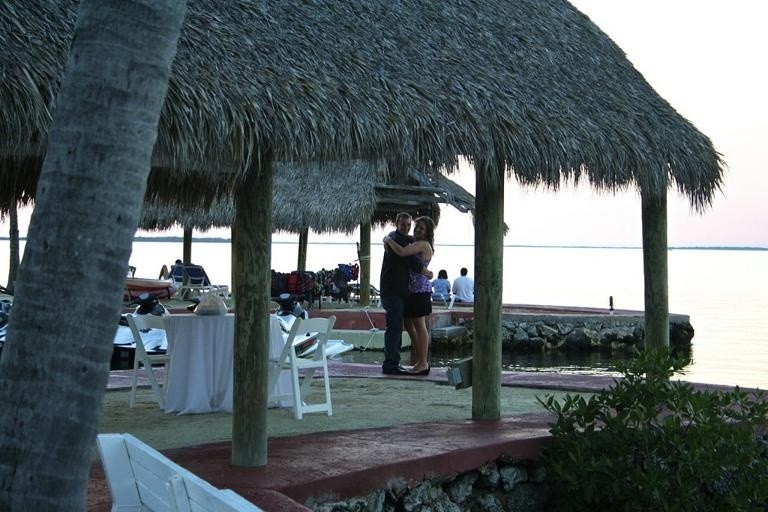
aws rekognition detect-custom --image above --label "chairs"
[267,315,339,421]
[125,312,168,412]
[168,264,230,302]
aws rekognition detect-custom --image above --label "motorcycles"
[141,296,201,366]
[0,298,13,352]
[111,291,171,370]
[269,293,318,358]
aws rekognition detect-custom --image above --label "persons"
[452,267,475,303]
[158,258,182,280]
[429,268,452,307]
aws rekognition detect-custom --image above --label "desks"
[161,313,279,412]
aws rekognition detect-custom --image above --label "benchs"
[93,432,267,512]
[349,282,381,305]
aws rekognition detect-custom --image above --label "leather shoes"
[383,366,429,375]
